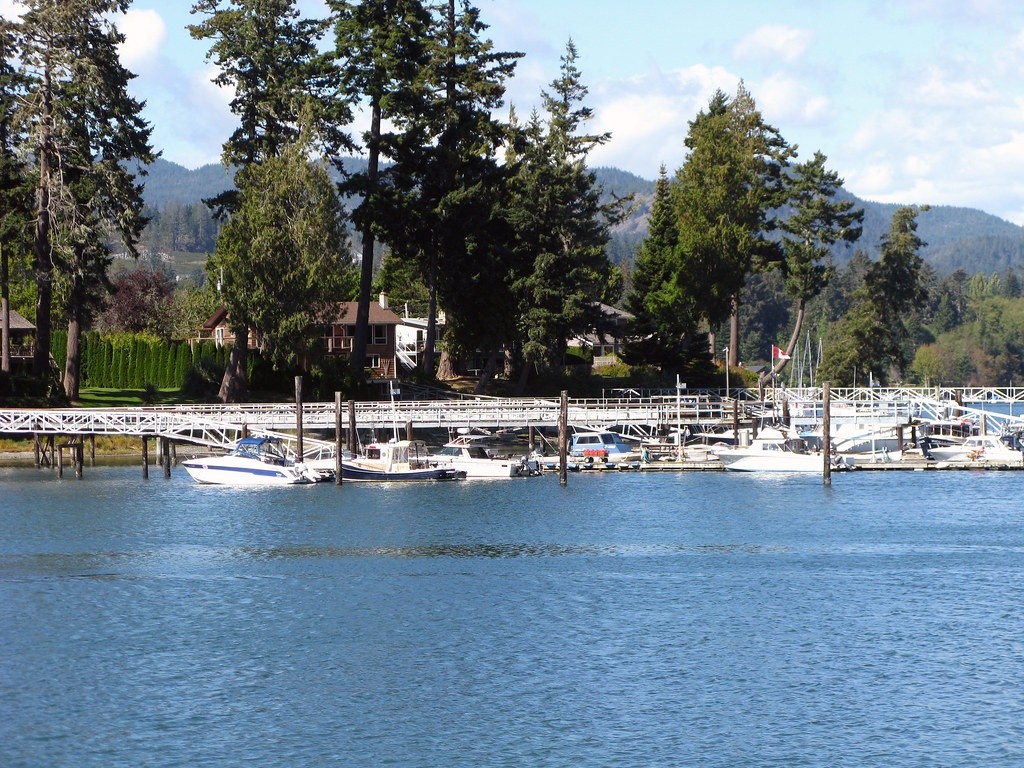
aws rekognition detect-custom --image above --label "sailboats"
[765,329,914,423]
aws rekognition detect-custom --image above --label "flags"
[773,346,791,359]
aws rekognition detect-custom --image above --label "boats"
[412,435,542,479]
[341,441,469,479]
[712,425,862,473]
[928,435,1023,464]
[660,444,721,460]
[531,430,661,466]
[917,418,980,460]
[1001,416,1024,451]
[182,436,335,487]
